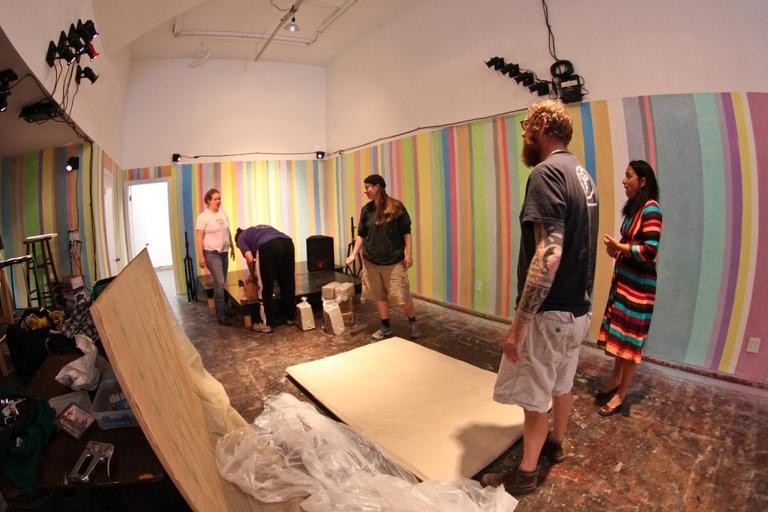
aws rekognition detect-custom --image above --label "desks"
[0,355,179,511]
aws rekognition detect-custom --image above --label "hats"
[364,174,386,187]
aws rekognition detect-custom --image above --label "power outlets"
[746,337,760,354]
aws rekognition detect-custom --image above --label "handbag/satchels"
[5,310,57,378]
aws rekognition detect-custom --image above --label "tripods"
[344,217,362,279]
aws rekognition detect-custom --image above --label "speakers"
[307,236,334,272]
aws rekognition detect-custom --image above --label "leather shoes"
[592,384,621,399]
[598,400,624,417]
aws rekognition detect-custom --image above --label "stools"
[23,233,59,310]
[0,254,42,319]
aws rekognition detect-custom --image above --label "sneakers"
[218,319,231,326]
[480,463,539,494]
[542,431,565,463]
[285,317,296,325]
[372,324,392,340]
[409,321,422,339]
[253,322,273,333]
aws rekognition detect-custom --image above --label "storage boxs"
[90,379,140,430]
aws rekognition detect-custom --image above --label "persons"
[593,160,662,416]
[345,175,422,340]
[480,99,599,495]
[196,188,235,325]
[235,225,296,333]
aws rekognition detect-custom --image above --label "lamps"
[0,69,18,112]
[485,56,550,96]
[283,5,299,32]
[65,156,79,172]
[46,19,100,84]
[172,154,181,163]
[317,151,324,159]
